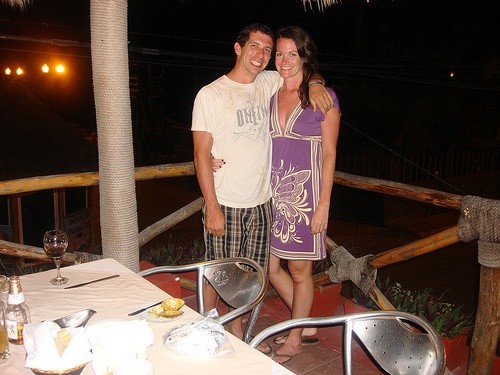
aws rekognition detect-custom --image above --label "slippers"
[268,347,303,364]
[273,334,321,346]
[242,334,272,355]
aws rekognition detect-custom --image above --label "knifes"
[64,274,121,290]
[128,297,162,316]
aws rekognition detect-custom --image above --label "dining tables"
[0,257,297,375]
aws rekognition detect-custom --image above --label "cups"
[42,309,97,333]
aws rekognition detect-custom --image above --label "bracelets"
[309,82,322,87]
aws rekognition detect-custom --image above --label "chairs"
[137,256,270,345]
[249,309,448,375]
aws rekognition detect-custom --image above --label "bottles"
[6,276,31,345]
[0,301,11,361]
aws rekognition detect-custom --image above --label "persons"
[191,21,335,355]
[210,26,340,364]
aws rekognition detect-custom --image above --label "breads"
[54,329,73,357]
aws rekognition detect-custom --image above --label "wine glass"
[43,230,71,285]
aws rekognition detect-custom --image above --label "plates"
[133,304,182,324]
